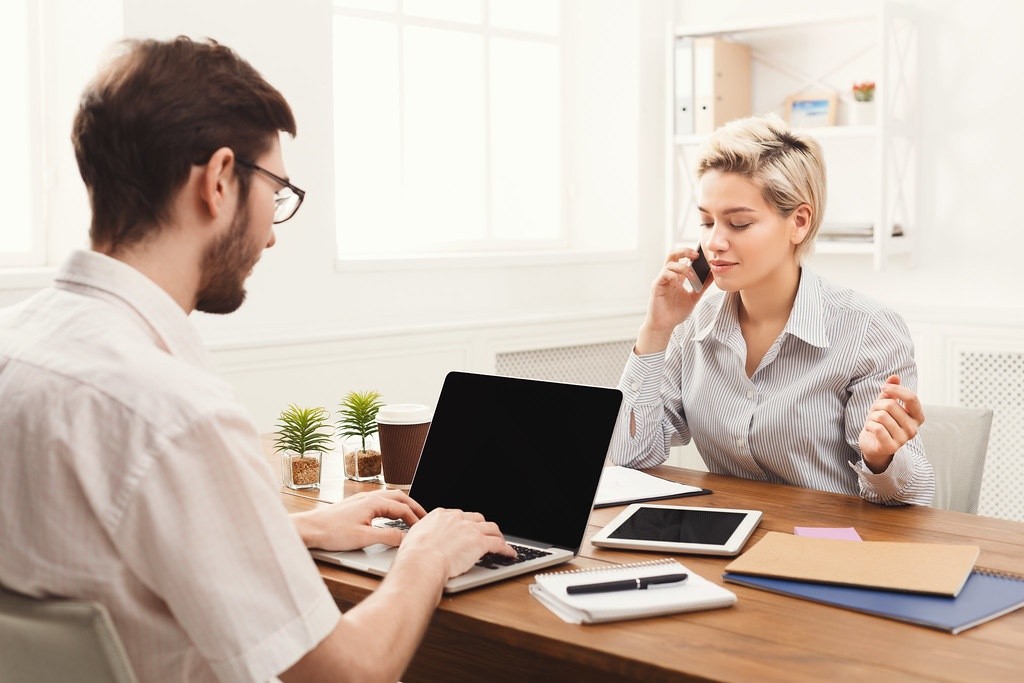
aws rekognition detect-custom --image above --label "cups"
[374,404,432,489]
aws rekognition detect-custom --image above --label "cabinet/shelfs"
[666,7,916,253]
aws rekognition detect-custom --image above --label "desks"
[280,465,1024,683]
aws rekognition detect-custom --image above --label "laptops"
[308,370,624,594]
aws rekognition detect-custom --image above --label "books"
[528,558,737,625]
[594,466,714,509]
[720,531,1024,636]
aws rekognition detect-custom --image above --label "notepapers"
[794,526,862,542]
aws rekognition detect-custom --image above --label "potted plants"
[335,391,387,482]
[273,402,337,489]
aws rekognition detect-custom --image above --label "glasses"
[191,146,306,224]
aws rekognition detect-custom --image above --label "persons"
[608,116,935,508]
[0,34,517,683]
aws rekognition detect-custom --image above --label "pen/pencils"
[567,574,690,594]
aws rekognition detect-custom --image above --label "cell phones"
[687,241,710,293]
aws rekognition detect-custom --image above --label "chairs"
[918,405,994,514]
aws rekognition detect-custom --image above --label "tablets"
[591,504,763,556]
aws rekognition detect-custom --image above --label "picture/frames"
[784,95,838,126]
